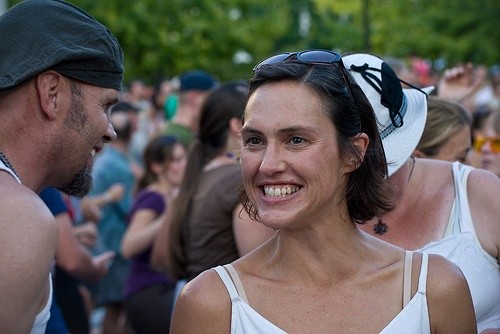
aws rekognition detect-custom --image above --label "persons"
[0,0,124,334]
[167,80,278,312]
[383,54,500,178]
[46,65,214,334]
[342,51,500,333]
[166,50,478,334]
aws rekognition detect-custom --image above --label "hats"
[175,72,219,91]
[341,53,436,179]
[1,0,123,93]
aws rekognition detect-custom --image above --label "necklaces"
[1,152,19,176]
[372,156,416,238]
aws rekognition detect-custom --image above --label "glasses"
[252,49,364,132]
